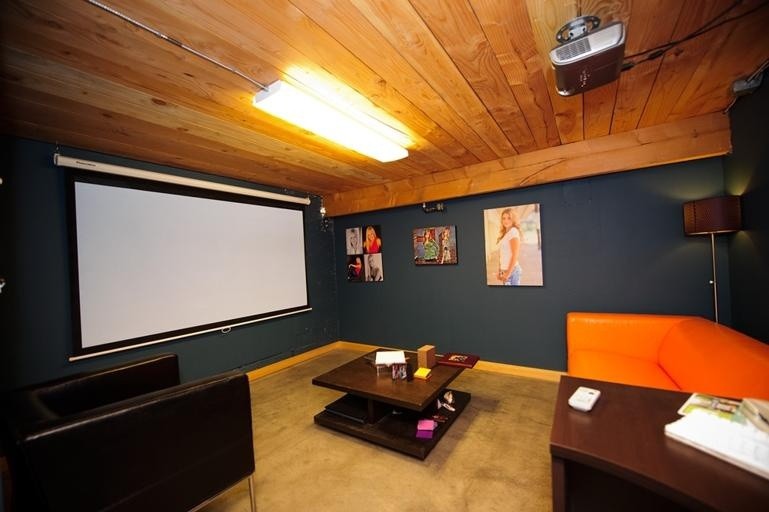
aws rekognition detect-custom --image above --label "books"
[678,392,757,430]
[325,394,387,425]
[739,396,769,433]
[668,409,769,482]
[436,353,481,370]
[375,350,406,364]
[367,355,413,370]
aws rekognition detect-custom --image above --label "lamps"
[683,195,743,324]
[252,80,409,165]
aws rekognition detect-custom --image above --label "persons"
[349,257,363,280]
[423,228,439,261]
[485,208,525,286]
[440,227,452,247]
[348,229,363,255]
[364,226,382,254]
[439,247,453,265]
[366,255,383,282]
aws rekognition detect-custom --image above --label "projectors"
[548,21,627,97]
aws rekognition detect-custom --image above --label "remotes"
[568,386,601,411]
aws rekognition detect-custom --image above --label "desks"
[550,375,769,512]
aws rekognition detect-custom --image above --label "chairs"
[1,353,257,512]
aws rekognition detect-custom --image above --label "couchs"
[566,312,769,402]
